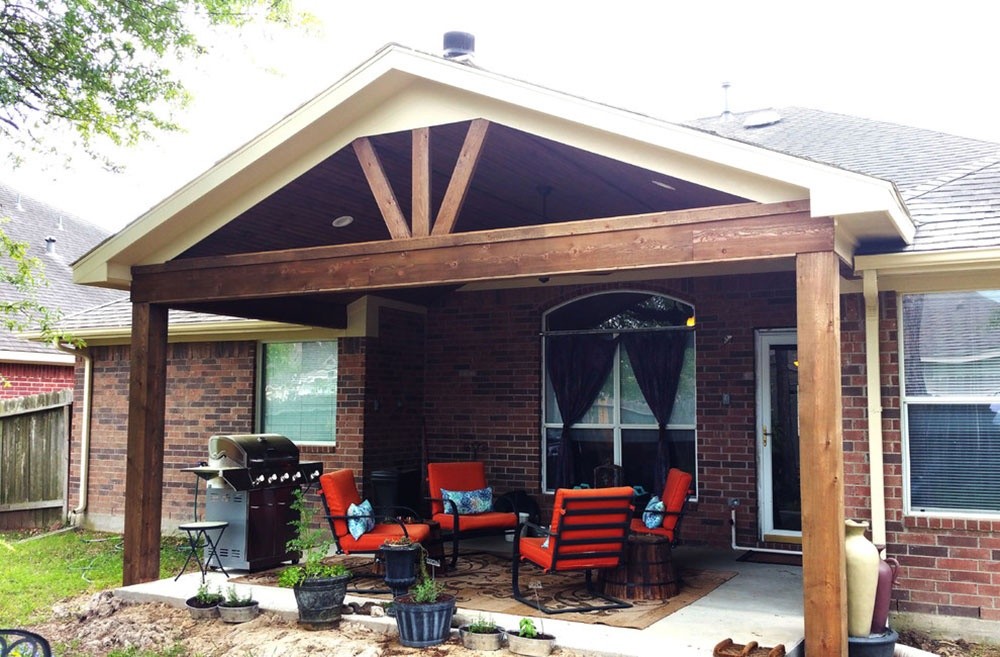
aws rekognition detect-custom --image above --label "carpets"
[224,544,742,635]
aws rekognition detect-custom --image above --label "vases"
[843,515,901,657]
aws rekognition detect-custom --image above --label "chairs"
[509,485,635,616]
[316,466,433,594]
[629,466,694,548]
[421,460,521,573]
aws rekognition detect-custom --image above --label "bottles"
[871,544,901,633]
[844,519,880,638]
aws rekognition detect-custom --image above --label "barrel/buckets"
[506,513,529,541]
[601,531,680,599]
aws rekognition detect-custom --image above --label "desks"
[173,520,230,584]
[595,532,683,602]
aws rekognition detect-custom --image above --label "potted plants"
[458,612,506,651]
[506,617,557,657]
[274,487,354,632]
[378,535,429,600]
[391,576,457,649]
[184,583,226,620]
[217,582,259,624]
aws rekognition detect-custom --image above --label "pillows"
[641,495,666,530]
[439,486,494,515]
[345,498,376,541]
